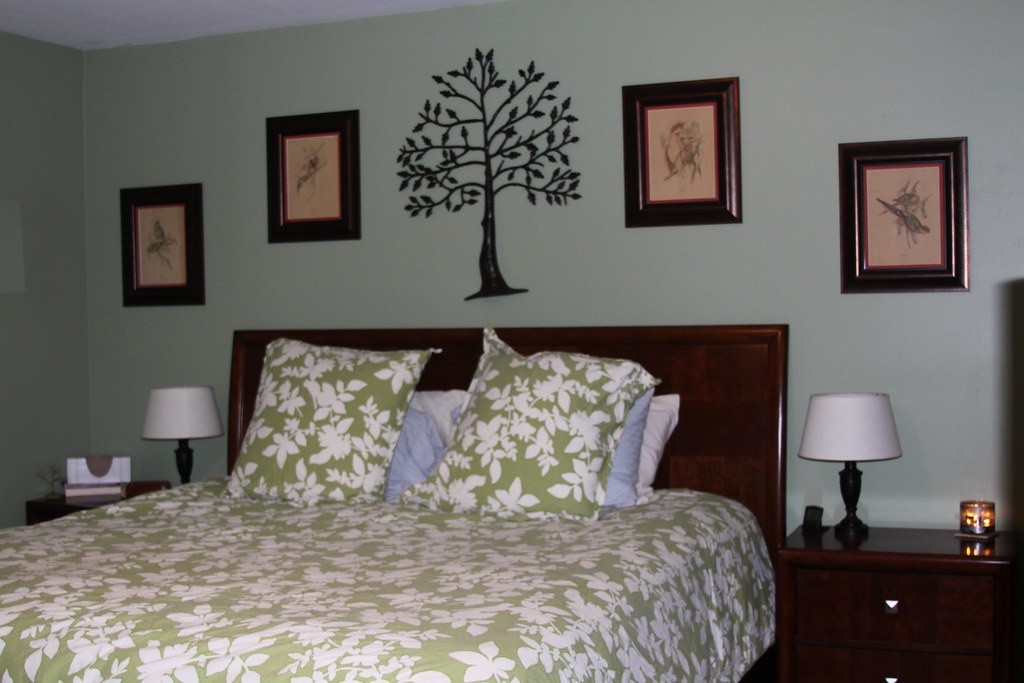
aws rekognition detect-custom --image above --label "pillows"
[638,391,681,508]
[400,327,660,518]
[382,408,451,503]
[605,388,654,509]
[408,390,464,445]
[220,335,441,505]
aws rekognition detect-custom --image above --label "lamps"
[798,390,905,541]
[142,387,223,486]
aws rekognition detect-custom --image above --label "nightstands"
[26,475,167,524]
[774,523,1017,683]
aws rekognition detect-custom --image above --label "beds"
[0,322,791,683]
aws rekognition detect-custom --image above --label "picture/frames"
[621,73,745,228]
[837,137,971,295]
[263,110,361,246]
[120,182,207,306]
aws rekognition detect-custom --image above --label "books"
[64,483,122,496]
